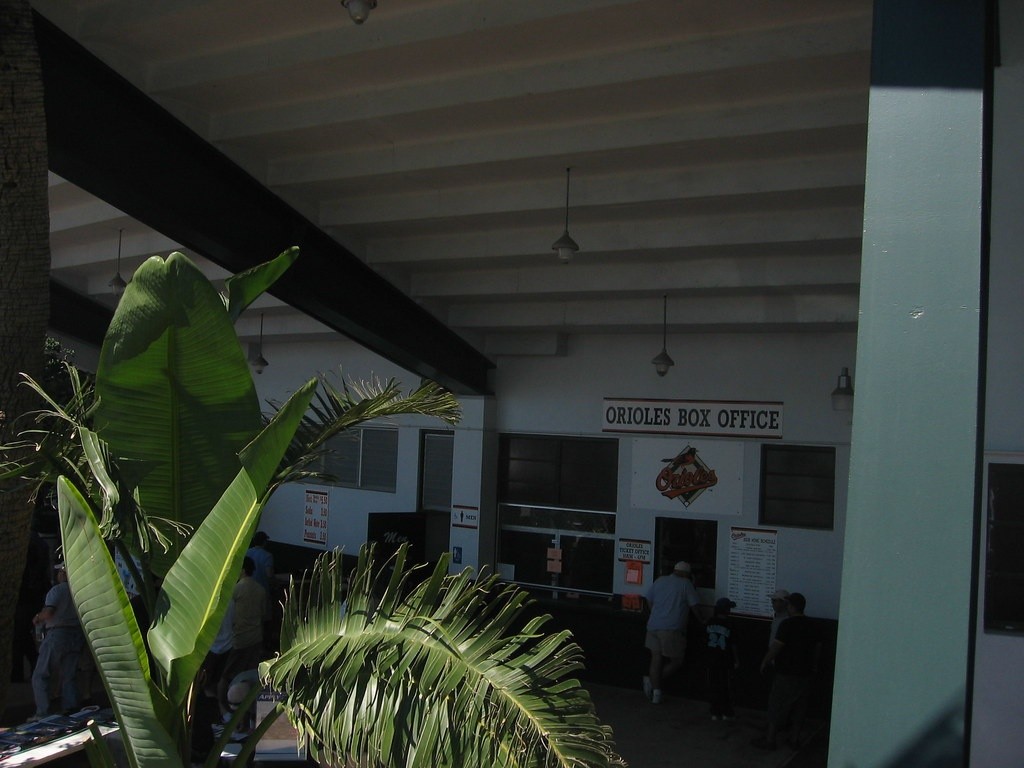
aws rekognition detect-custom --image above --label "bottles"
[36,623,44,641]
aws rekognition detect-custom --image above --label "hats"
[766,590,790,602]
[783,592,806,611]
[54,561,65,571]
[674,561,690,573]
[715,597,737,611]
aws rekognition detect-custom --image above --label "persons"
[25,561,82,722]
[10,518,52,684]
[749,592,815,751]
[187,532,420,768]
[706,597,740,723]
[640,559,710,705]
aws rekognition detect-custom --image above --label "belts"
[45,626,81,631]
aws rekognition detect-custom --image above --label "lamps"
[554,167,580,264]
[107,228,128,294]
[652,300,674,375]
[831,365,855,411]
[247,314,270,374]
[339,0,377,25]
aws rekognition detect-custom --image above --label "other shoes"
[651,695,668,704]
[711,716,719,720]
[27,715,39,723]
[785,737,799,750]
[749,736,777,751]
[722,715,735,721]
[643,676,653,700]
